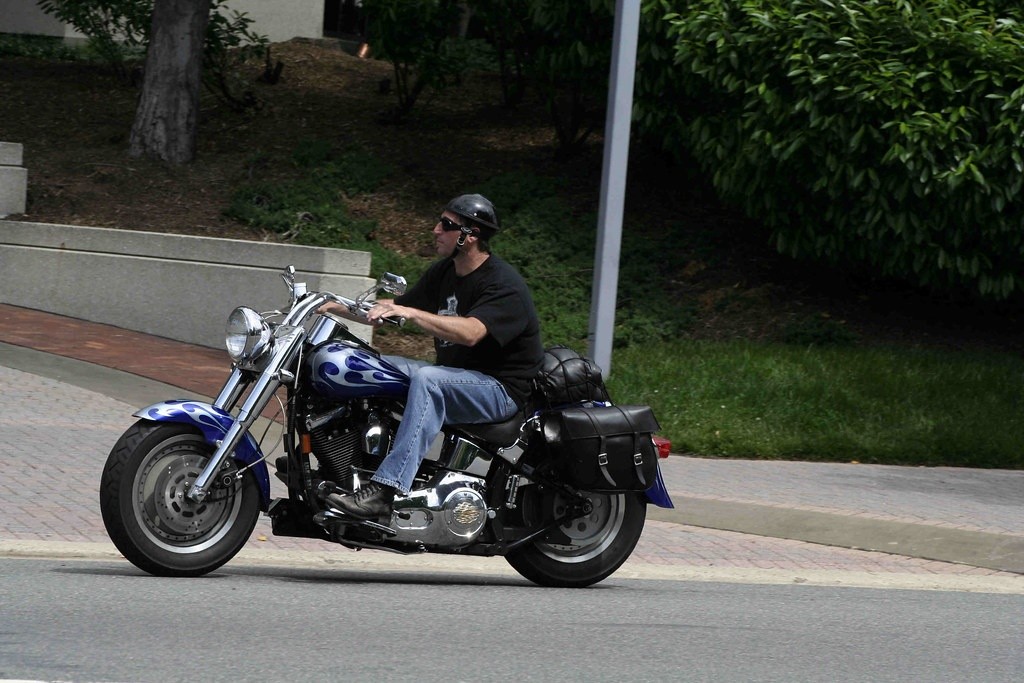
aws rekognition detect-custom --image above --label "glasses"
[439,216,475,235]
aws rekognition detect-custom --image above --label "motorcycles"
[99,265,675,589]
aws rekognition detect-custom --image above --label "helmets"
[445,193,501,234]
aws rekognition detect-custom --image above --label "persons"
[311,195,546,519]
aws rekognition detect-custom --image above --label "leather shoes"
[327,482,397,527]
[275,455,321,482]
